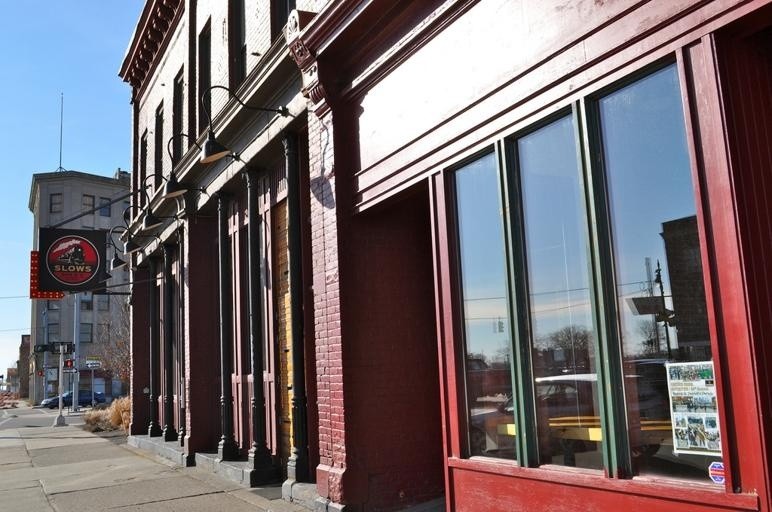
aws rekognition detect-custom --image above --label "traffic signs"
[86,361,102,368]
[87,356,102,360]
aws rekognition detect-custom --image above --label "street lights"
[654,259,673,360]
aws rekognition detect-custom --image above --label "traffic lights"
[38,370,44,376]
[65,359,73,367]
[500,322,504,332]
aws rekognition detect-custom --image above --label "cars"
[467,355,683,461]
[41,389,107,409]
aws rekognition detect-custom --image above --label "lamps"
[192,80,300,161]
[109,223,135,273]
[121,204,147,255]
[139,175,191,232]
[160,133,250,203]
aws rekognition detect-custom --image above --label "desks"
[492,412,675,469]
